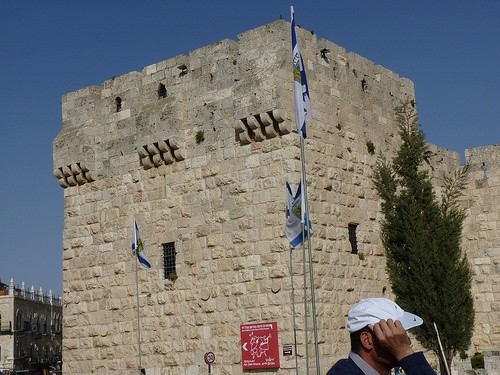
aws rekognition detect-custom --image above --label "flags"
[284,179,313,250]
[132,220,151,269]
[289,5,313,138]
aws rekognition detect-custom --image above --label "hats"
[346,297,422,333]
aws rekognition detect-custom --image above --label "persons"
[325,297,437,375]
